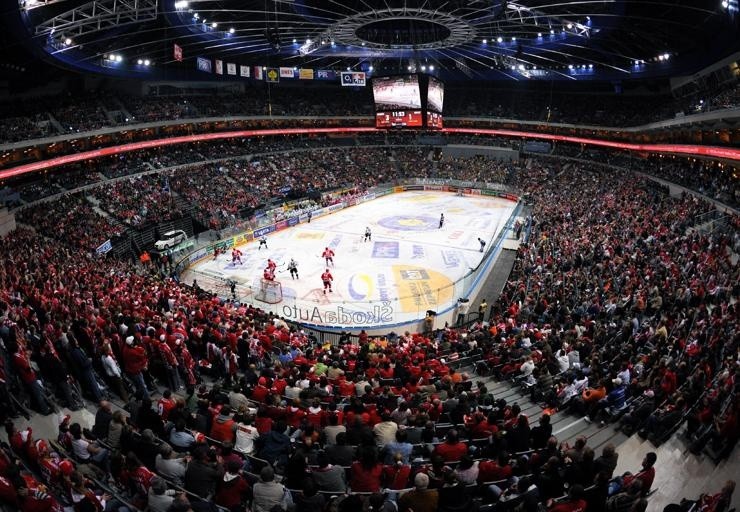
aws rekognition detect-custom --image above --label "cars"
[154,230,187,251]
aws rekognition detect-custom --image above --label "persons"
[438,212,446,230]
[0,233,289,325]
[488,205,739,325]
[456,297,471,327]
[363,226,372,243]
[477,298,487,324]
[0,325,740,512]
[320,268,335,294]
[0,83,279,233]
[424,312,436,332]
[279,84,740,205]
[321,247,336,267]
[477,236,487,252]
[285,257,300,280]
[275,201,323,224]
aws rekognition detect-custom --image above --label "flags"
[170,43,369,88]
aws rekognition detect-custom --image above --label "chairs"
[0,290,740,512]
